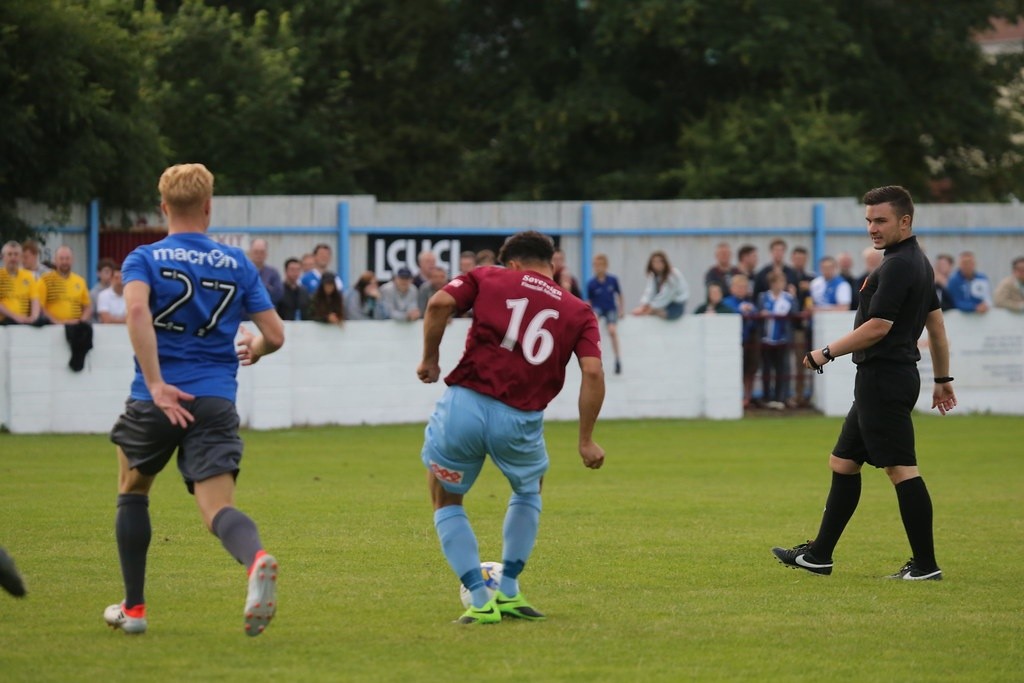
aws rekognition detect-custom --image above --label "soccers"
[458,559,520,612]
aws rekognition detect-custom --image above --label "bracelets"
[934,376,954,383]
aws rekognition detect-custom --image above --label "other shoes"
[0,549,25,597]
[768,400,786,411]
[614,362,621,373]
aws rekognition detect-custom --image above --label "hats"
[398,267,412,278]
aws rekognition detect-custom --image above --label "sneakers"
[245,550,278,636]
[771,541,834,575]
[881,557,943,582]
[452,599,502,624]
[104,599,147,634]
[494,591,547,621]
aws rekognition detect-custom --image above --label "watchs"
[821,344,835,362]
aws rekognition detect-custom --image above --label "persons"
[416,231,605,625]
[104,163,285,637]
[0,230,1024,409]
[772,186,958,582]
